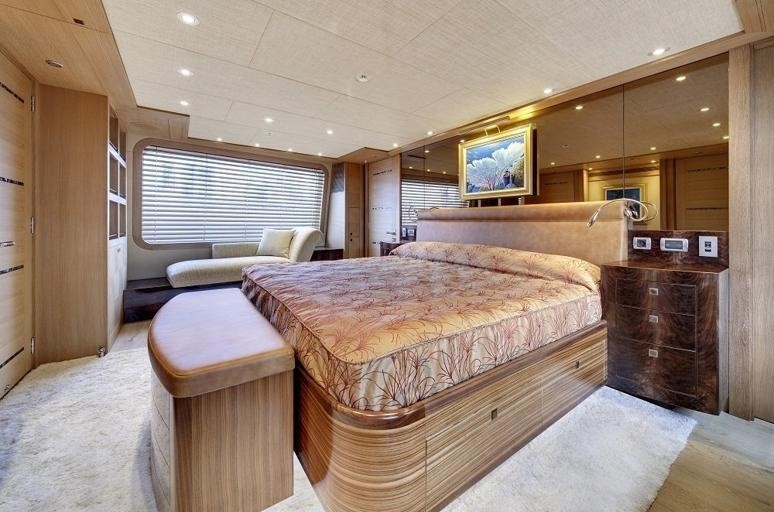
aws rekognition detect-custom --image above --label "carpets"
[1,346,697,510]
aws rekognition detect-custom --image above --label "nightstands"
[379,239,401,252]
[603,257,730,417]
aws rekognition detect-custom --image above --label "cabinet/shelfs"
[333,163,365,256]
[36,81,130,371]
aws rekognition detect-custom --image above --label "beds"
[241,199,628,512]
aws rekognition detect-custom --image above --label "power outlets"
[698,236,717,258]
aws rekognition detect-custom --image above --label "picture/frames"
[453,122,542,201]
[603,182,648,225]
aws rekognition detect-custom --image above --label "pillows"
[254,227,295,256]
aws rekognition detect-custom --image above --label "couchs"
[161,226,324,289]
